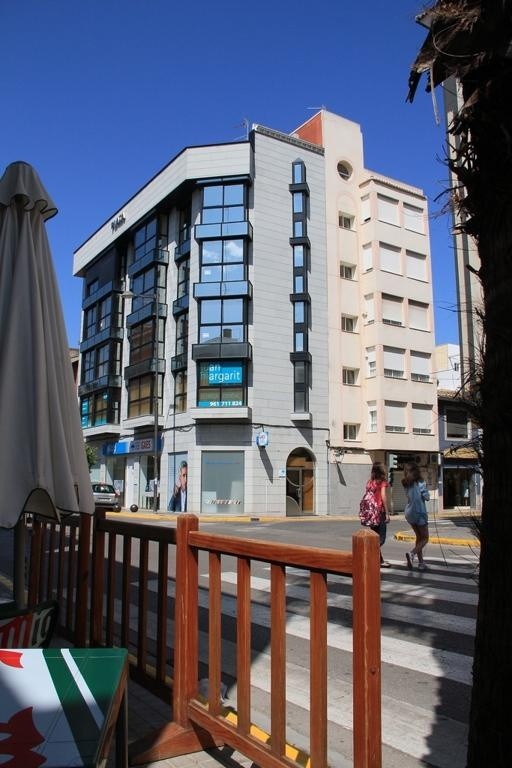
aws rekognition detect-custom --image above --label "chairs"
[0,600,58,648]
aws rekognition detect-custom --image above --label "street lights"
[121,291,160,513]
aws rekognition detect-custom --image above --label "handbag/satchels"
[405,503,416,521]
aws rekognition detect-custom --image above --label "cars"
[91,482,120,511]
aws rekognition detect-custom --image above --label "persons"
[400,461,430,571]
[168,460,187,512]
[366,461,392,567]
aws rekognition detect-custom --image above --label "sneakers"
[381,562,391,568]
[406,552,429,570]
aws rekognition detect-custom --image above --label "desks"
[0,646,128,768]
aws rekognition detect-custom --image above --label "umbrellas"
[0,161,97,612]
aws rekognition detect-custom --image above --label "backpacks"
[360,480,382,527]
